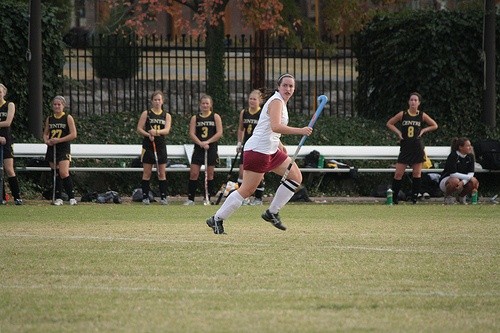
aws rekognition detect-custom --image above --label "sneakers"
[70,198,77,205]
[51,199,64,205]
[261,209,286,230]
[206,216,224,234]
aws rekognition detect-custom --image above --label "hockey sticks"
[212,151,239,205]
[51,144,57,205]
[151,141,161,181]
[280,95,329,184]
[0,143,4,204]
[204,149,210,206]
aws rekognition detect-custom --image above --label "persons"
[137,91,171,205]
[236,90,265,205]
[384,92,438,205]
[438,137,479,206]
[181,96,223,205]
[206,73,313,235]
[43,95,77,206]
[0,84,22,205]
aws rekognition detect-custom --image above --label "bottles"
[317,155,325,168]
[386,186,393,205]
[227,155,232,168]
[471,188,478,205]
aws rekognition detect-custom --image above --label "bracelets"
[156,130,159,136]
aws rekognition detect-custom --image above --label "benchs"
[0,145,484,191]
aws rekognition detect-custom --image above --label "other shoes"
[183,200,194,205]
[457,194,467,205]
[161,199,168,204]
[15,198,23,205]
[242,200,247,205]
[446,194,456,204]
[143,198,150,204]
[249,198,263,205]
